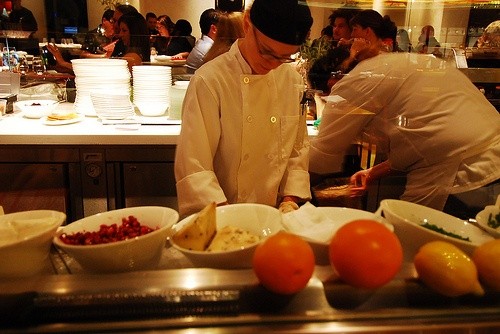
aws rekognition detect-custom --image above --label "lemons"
[472,239,500,294]
[414,241,484,297]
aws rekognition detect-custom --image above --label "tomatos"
[252,232,315,294]
[329,220,403,289]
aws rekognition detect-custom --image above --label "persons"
[396,29,415,52]
[0,0,38,55]
[197,11,245,69]
[294,5,404,97]
[46,5,196,78]
[415,25,441,54]
[185,8,222,74]
[307,45,500,220]
[174,0,313,220]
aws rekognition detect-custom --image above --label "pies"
[171,201,268,252]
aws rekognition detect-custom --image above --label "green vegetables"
[487,210,500,229]
[420,223,470,241]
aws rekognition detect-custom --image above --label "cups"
[17,55,44,74]
[60,38,73,46]
[290,52,307,65]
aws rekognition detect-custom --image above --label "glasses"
[251,30,297,63]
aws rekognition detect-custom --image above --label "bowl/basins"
[52,206,179,273]
[281,206,394,259]
[169,202,283,270]
[476,207,500,237]
[311,178,364,206]
[0,209,67,278]
[132,65,173,116]
[380,199,495,261]
[14,100,59,118]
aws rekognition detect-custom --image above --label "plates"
[153,56,170,61]
[70,58,137,125]
[168,84,188,121]
[38,110,85,125]
[171,60,187,64]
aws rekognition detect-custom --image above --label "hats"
[249,0,313,46]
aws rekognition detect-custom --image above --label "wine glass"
[3,47,18,73]
